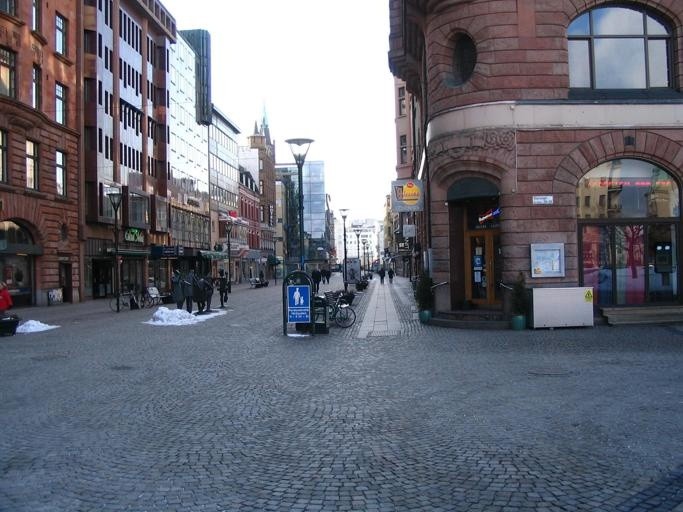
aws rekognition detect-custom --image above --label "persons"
[325,269,331,284]
[311,268,316,282]
[387,268,393,284]
[320,268,326,284]
[379,268,385,284]
[171,269,213,315]
[259,270,263,281]
[315,269,321,293]
[0,281,13,319]
[347,268,359,284]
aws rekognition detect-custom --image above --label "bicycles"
[108,284,154,312]
[329,289,356,329]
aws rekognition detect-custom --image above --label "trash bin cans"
[313,296,329,334]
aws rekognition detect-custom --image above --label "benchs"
[249,278,261,288]
[145,286,168,306]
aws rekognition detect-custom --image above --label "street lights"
[106,191,124,312]
[271,234,277,285]
[284,138,317,270]
[339,208,351,292]
[223,215,233,293]
[351,219,373,277]
